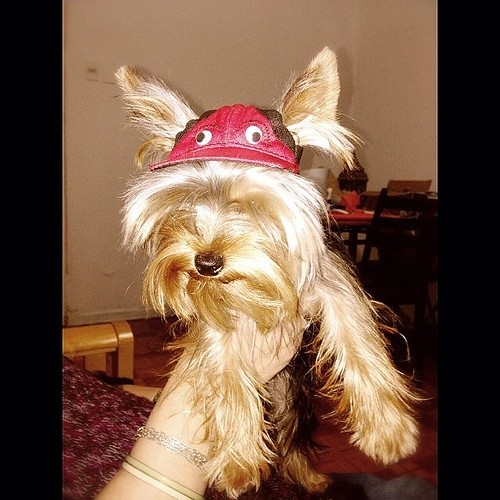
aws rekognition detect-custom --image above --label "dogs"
[111,45,434,500]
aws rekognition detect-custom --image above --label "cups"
[308,168,328,194]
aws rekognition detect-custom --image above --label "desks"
[327,205,403,266]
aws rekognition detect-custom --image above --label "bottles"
[337,151,369,193]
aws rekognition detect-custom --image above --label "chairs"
[358,188,438,374]
[386,180,432,192]
